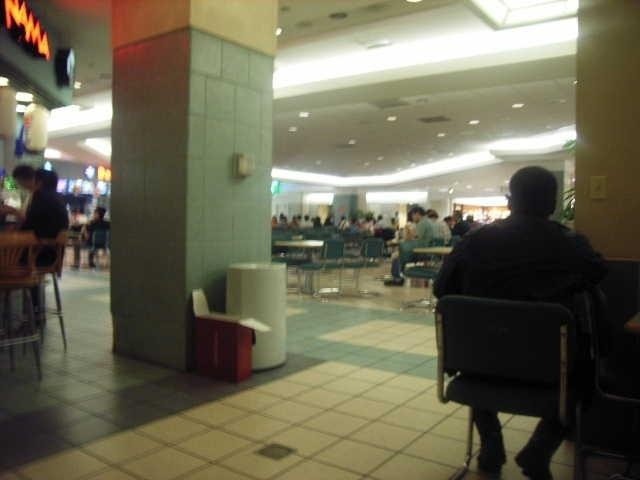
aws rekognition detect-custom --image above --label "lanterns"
[24,103,48,154]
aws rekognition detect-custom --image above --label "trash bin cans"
[224,261,287,373]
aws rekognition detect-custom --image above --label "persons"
[3,165,68,267]
[272,207,471,285]
[71,207,110,267]
[433,166,640,480]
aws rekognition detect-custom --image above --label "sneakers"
[477,443,506,470]
[385,277,404,285]
[515,445,552,479]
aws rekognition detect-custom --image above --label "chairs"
[433,291,640,480]
[86,228,110,270]
[270,224,463,310]
[0,226,69,381]
